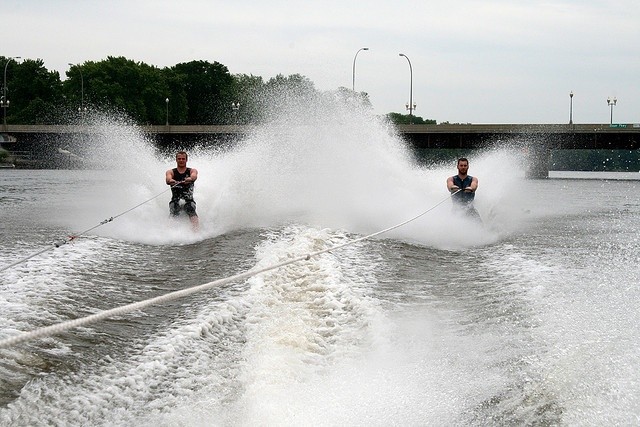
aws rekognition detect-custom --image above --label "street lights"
[607,96,617,127]
[569,90,573,125]
[166,98,169,126]
[68,62,87,126]
[232,102,240,125]
[398,53,417,115]
[0,56,21,125]
[352,48,368,94]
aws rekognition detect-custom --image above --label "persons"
[446,157,485,229]
[164,149,200,237]
